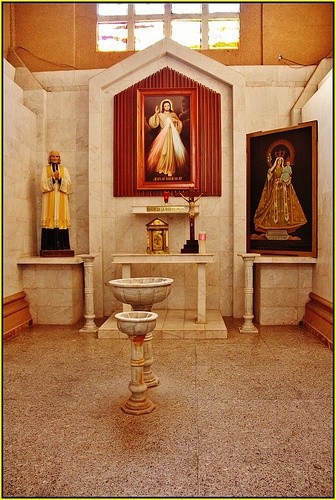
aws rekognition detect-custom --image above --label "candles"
[163,192,170,204]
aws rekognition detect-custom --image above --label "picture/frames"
[135,87,201,190]
[246,120,320,257]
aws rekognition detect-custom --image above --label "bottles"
[198,228,207,254]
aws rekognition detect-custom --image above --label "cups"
[164,192,169,206]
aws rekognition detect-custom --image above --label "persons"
[39,150,73,252]
